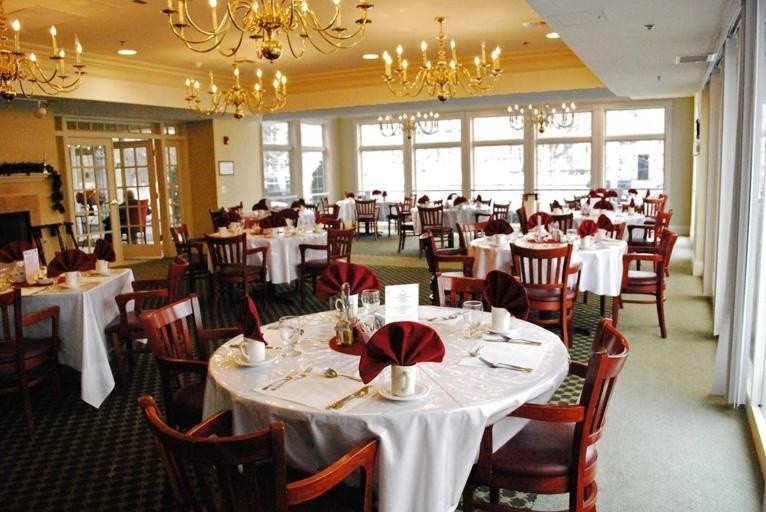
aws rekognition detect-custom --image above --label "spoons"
[468,344,487,357]
[425,306,462,323]
[499,332,542,348]
[321,367,362,383]
[334,386,370,410]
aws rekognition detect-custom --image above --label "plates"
[376,386,431,401]
[234,357,275,367]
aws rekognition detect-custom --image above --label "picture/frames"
[218,160,234,176]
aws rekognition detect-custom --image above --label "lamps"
[506,101,576,134]
[0,15,85,114]
[377,112,439,137]
[161,0,374,63]
[381,15,505,103]
[182,64,288,123]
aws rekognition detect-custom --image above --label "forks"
[262,366,313,391]
[479,357,527,373]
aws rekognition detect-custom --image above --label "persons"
[105,189,151,242]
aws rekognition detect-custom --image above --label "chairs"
[354,198,380,242]
[476,199,491,208]
[419,230,475,306]
[548,213,575,233]
[296,227,355,308]
[449,276,520,308]
[515,206,530,235]
[643,193,668,240]
[137,395,378,512]
[393,202,415,253]
[321,195,340,217]
[462,317,629,512]
[103,254,190,394]
[138,293,241,429]
[475,203,510,231]
[613,227,679,339]
[386,197,412,238]
[627,211,674,278]
[0,288,63,444]
[170,223,204,272]
[418,205,454,260]
[204,232,268,323]
[118,199,148,246]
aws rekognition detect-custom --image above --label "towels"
[316,262,382,301]
[94,239,115,263]
[528,212,552,228]
[477,195,481,202]
[485,220,514,236]
[259,214,287,228]
[359,322,446,384]
[454,196,467,206]
[596,214,614,231]
[578,220,598,238]
[482,270,529,321]
[593,201,613,210]
[242,295,268,345]
[0,241,35,263]
[47,249,92,278]
[418,195,429,203]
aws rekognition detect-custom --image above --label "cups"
[360,289,381,316]
[335,294,359,319]
[390,364,418,396]
[462,301,484,341]
[95,259,110,275]
[239,337,266,362]
[278,315,302,362]
[490,306,510,330]
[65,271,83,288]
